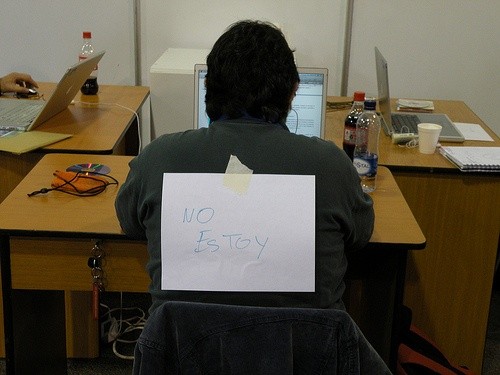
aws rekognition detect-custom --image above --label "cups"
[417,122,443,154]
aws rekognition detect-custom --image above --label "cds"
[65,163,111,175]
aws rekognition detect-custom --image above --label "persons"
[114,19,412,373]
[0,72,39,95]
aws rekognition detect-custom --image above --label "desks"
[0,83,500,375]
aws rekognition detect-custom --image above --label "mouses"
[16,88,38,97]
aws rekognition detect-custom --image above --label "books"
[440,146,500,172]
[397,99,434,112]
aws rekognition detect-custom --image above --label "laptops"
[0,50,106,132]
[372,44,465,142]
[191,63,329,141]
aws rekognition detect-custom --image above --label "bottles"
[343,90,370,161]
[353,99,381,193]
[79,32,99,95]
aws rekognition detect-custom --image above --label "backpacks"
[392,323,479,375]
